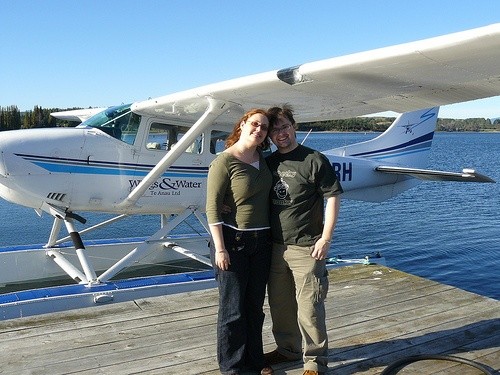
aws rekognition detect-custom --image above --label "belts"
[224,228,270,240]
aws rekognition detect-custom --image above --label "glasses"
[269,124,293,135]
[246,118,268,131]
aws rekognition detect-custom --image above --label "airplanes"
[0,23,500,321]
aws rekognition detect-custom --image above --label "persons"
[205,110,273,375]
[261,107,344,375]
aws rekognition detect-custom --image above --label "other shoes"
[302,369,321,375]
[253,361,274,375]
[262,350,290,363]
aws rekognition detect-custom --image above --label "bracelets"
[216,249,225,253]
[322,238,332,245]
